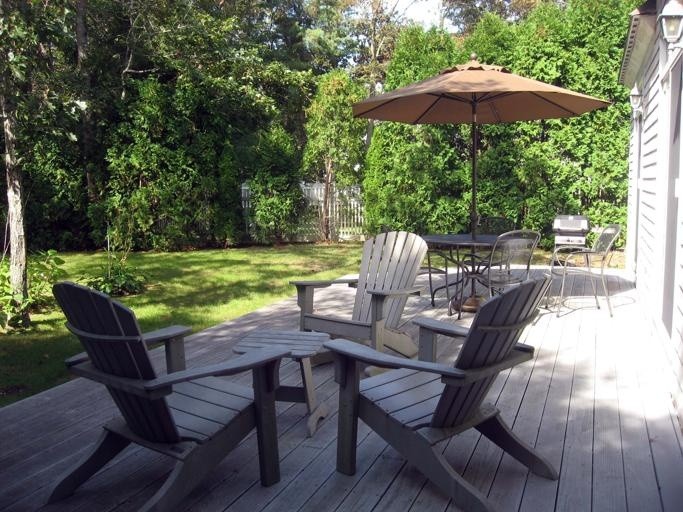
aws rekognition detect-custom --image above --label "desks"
[421,235,513,316]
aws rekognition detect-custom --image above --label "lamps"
[629,81,644,120]
[657,0,683,51]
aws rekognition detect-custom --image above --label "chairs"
[322,276,559,511]
[45,282,292,511]
[381,224,450,306]
[547,215,590,276]
[545,225,621,317]
[458,230,541,319]
[289,230,428,377]
[455,217,516,301]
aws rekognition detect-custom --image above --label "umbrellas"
[344,52,614,312]
